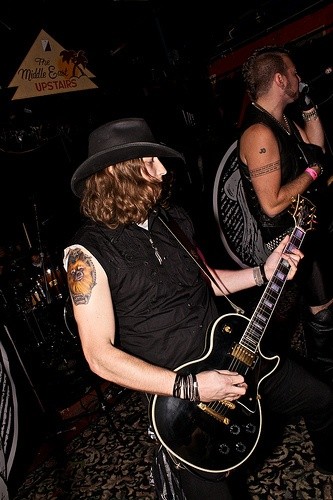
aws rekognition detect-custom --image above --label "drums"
[21,266,63,314]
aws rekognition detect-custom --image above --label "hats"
[69,117,186,197]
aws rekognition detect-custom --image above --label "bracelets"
[306,167,317,180]
[302,110,318,119]
[252,262,269,286]
[173,372,201,403]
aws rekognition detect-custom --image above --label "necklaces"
[252,102,309,164]
[136,223,163,265]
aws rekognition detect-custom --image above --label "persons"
[61,118,304,499]
[237,45,333,368]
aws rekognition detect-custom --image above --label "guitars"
[146,193,319,479]
